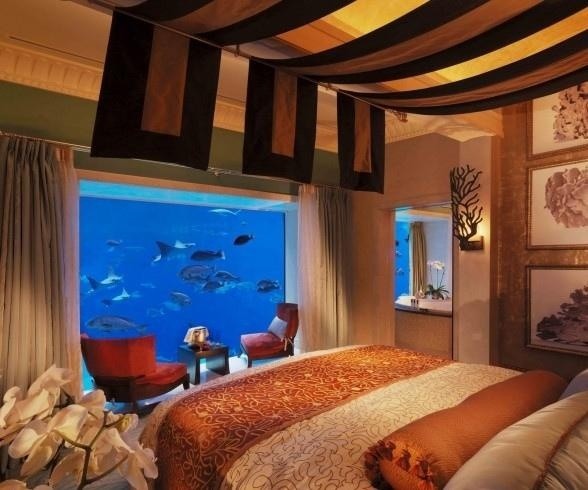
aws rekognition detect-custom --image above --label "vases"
[431,291,440,299]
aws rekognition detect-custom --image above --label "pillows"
[442,387,586,490]
[361,371,566,490]
[268,315,289,342]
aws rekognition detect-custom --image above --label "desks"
[175,341,229,387]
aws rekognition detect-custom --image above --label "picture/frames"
[523,80,588,161]
[527,156,587,250]
[523,263,588,358]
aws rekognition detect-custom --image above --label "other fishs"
[395,231,414,280]
[79,207,280,334]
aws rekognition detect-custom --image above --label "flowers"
[0,362,161,490]
[422,259,450,300]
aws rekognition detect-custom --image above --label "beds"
[135,344,586,490]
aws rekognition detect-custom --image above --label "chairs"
[79,330,192,411]
[239,302,301,371]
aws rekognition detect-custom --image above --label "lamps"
[447,162,489,252]
